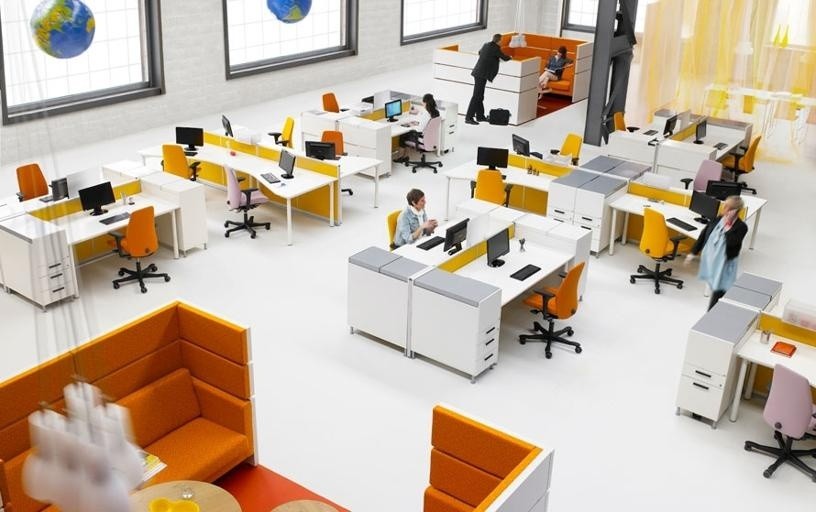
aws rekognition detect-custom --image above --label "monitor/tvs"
[278,149,295,179]
[222,115,233,137]
[444,218,469,255]
[688,189,720,224]
[512,133,529,157]
[175,127,203,150]
[477,146,508,171]
[305,141,335,160]
[487,229,510,266]
[362,96,374,104]
[384,99,402,122]
[51,178,68,201]
[663,115,677,138]
[693,121,706,144]
[707,180,741,202]
[79,181,115,214]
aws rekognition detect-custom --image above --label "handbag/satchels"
[509,36,527,48]
[490,108,509,124]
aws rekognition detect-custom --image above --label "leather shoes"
[465,119,479,125]
[477,115,487,121]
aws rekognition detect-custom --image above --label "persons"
[394,94,438,165]
[682,193,748,315]
[538,44,575,100]
[390,188,438,249]
[464,34,512,128]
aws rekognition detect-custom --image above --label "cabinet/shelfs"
[546,153,652,259]
[675,275,783,429]
[0,215,76,312]
[440,101,459,156]
[346,245,503,384]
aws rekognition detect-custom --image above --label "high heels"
[402,156,409,162]
[393,157,402,164]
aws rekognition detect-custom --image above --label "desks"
[340,102,445,157]
[444,158,556,222]
[632,119,746,179]
[388,217,576,298]
[728,331,816,426]
[141,125,384,248]
[611,182,766,297]
[24,177,183,259]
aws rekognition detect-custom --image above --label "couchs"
[2,368,252,511]
[511,46,576,98]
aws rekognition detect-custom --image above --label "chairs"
[111,206,173,293]
[387,210,405,250]
[15,163,51,203]
[518,261,586,356]
[743,364,816,482]
[322,92,346,113]
[266,117,296,150]
[469,169,514,208]
[405,117,444,174]
[677,136,763,195]
[610,112,637,133]
[550,134,583,164]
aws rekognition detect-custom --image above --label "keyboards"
[184,152,197,156]
[416,236,446,251]
[334,157,341,161]
[714,143,729,150]
[509,264,541,280]
[667,217,696,231]
[261,173,280,183]
[643,130,658,136]
[39,195,54,202]
[99,212,131,224]
[400,121,419,127]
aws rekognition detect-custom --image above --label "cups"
[149,497,200,512]
[127,198,135,203]
[760,330,771,344]
[120,190,127,205]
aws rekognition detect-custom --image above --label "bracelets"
[726,215,734,228]
